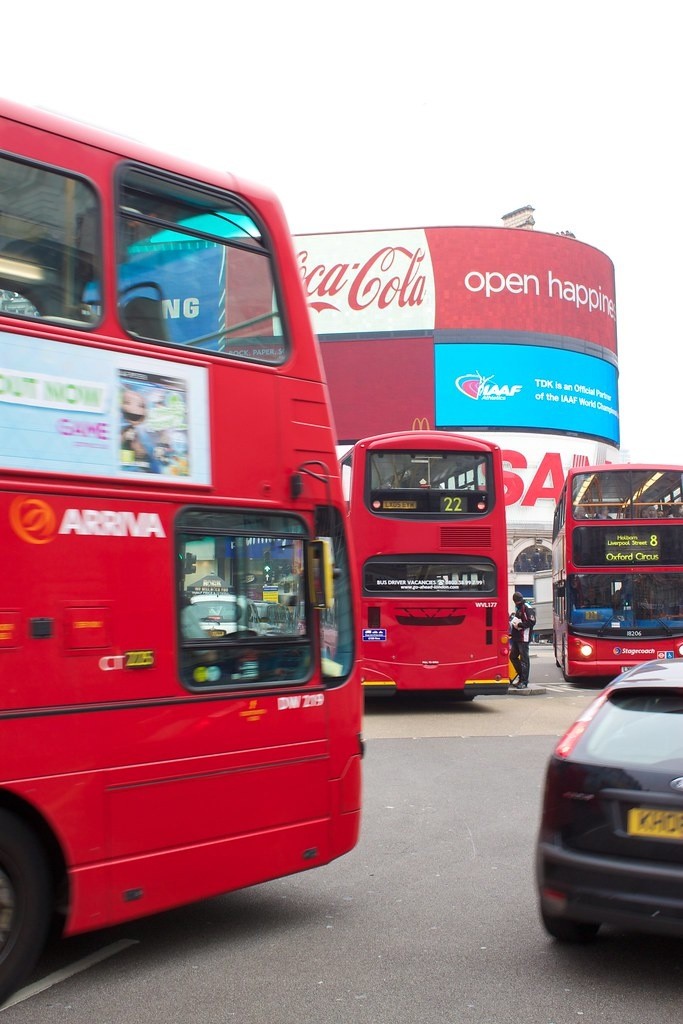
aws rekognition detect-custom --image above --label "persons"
[574,507,588,519]
[640,502,683,518]
[595,506,612,519]
[510,592,536,689]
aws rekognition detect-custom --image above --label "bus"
[0,98,367,1013]
[552,463,682,682]
[336,431,510,711]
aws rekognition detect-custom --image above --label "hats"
[513,592,523,601]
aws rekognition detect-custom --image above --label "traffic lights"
[185,552,197,574]
[262,548,272,583]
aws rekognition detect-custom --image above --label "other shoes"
[517,682,527,689]
[513,682,521,687]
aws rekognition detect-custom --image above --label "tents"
[187,569,234,594]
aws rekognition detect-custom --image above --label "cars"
[536,658,683,951]
[189,593,308,640]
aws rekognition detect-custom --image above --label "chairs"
[118,280,169,341]
